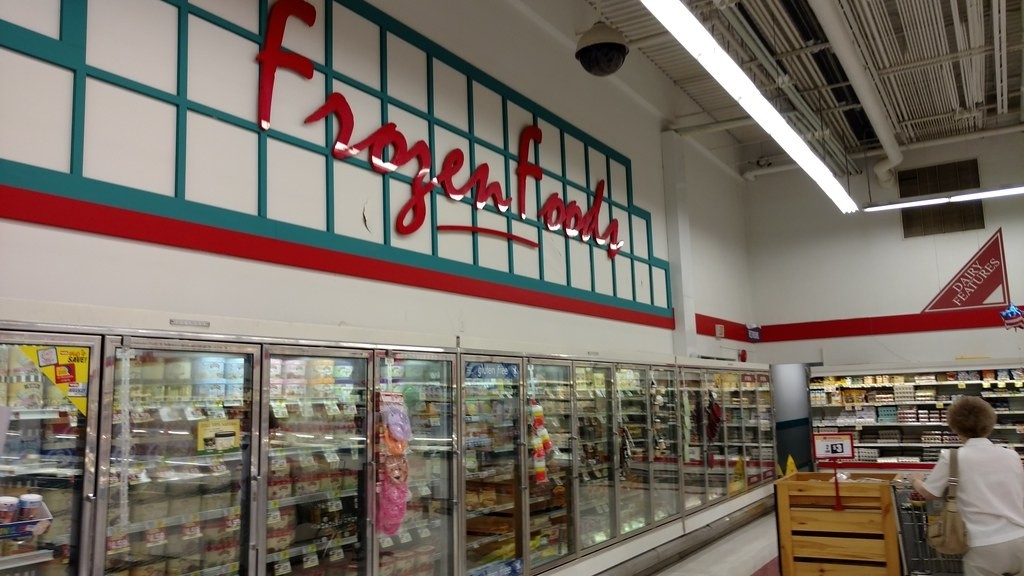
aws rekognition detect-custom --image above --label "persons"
[907,396,1024,576]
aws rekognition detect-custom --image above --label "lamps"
[862,142,1024,211]
[640,0,860,215]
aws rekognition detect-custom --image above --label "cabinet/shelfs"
[809,365,1024,469]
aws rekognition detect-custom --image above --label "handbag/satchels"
[927,449,967,554]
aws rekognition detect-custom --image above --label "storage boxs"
[816,391,822,404]
[810,391,816,404]
[821,390,828,404]
[774,471,903,576]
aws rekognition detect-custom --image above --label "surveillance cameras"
[575,21,629,77]
